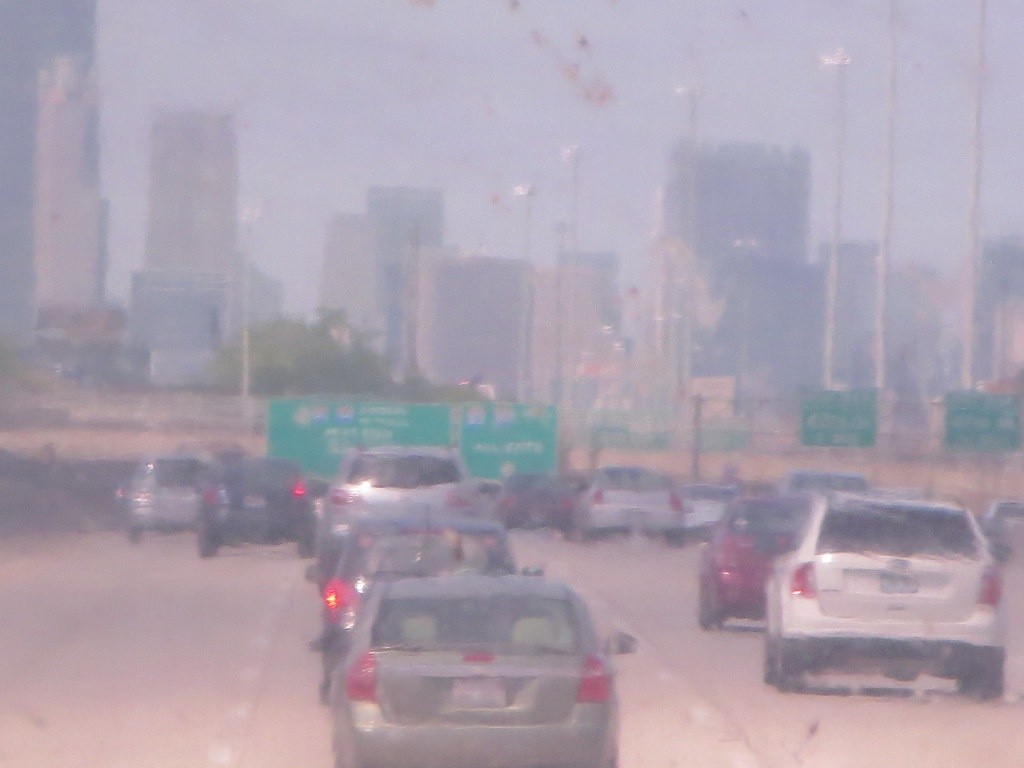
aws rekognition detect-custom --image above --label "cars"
[319,518,515,701]
[758,488,1005,698]
[313,445,507,536]
[325,575,638,768]
[113,449,213,543]
[501,466,1023,563]
[696,497,795,629]
[199,456,314,557]
